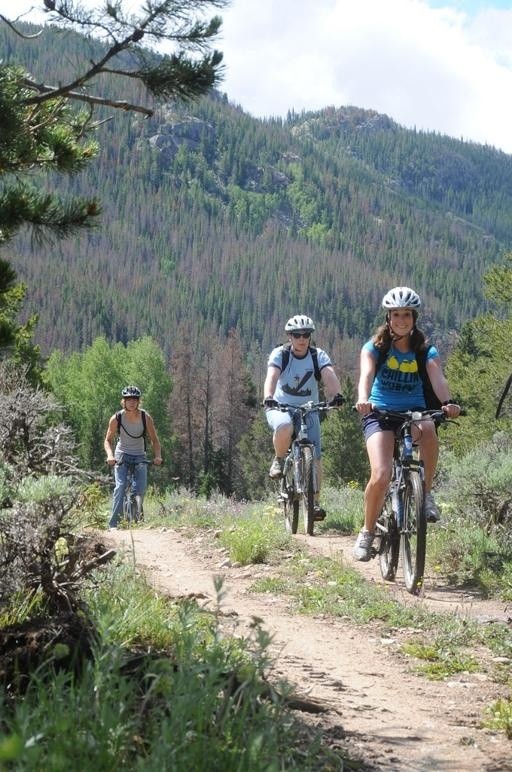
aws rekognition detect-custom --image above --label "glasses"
[291,332,311,339]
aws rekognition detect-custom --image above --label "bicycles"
[104,455,163,525]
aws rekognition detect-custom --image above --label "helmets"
[120,386,141,398]
[380,286,422,310]
[284,315,316,333]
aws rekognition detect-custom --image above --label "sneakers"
[314,502,326,517]
[269,455,285,479]
[424,492,440,520]
[352,527,376,561]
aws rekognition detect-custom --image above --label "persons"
[350,287,462,561]
[262,314,344,520]
[103,385,163,529]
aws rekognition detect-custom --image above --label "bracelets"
[442,399,459,404]
